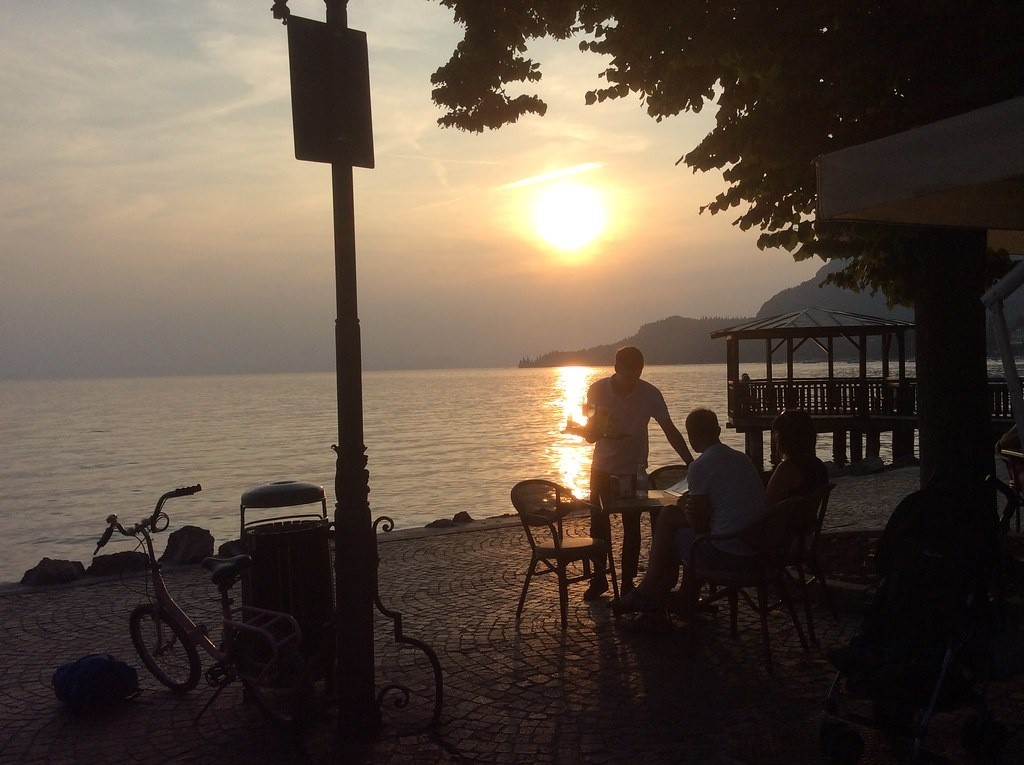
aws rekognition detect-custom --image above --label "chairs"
[995,433,1024,533]
[794,483,841,645]
[508,478,621,630]
[682,494,812,665]
[646,463,688,491]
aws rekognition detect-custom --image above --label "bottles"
[567,415,572,428]
[636,463,649,498]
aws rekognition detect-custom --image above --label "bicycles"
[93,484,314,731]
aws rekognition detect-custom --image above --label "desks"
[1001,447,1024,531]
[572,486,717,623]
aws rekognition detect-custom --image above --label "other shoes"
[662,592,692,621]
[583,577,609,602]
[622,584,634,598]
[610,597,662,613]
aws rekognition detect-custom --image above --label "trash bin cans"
[240,480,336,658]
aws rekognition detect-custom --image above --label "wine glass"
[583,394,596,429]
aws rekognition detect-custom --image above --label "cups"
[598,406,610,433]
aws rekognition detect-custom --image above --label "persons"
[612,409,764,613]
[571,347,694,607]
[765,409,828,557]
[739,373,759,412]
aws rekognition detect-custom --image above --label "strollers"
[817,468,1020,765]
